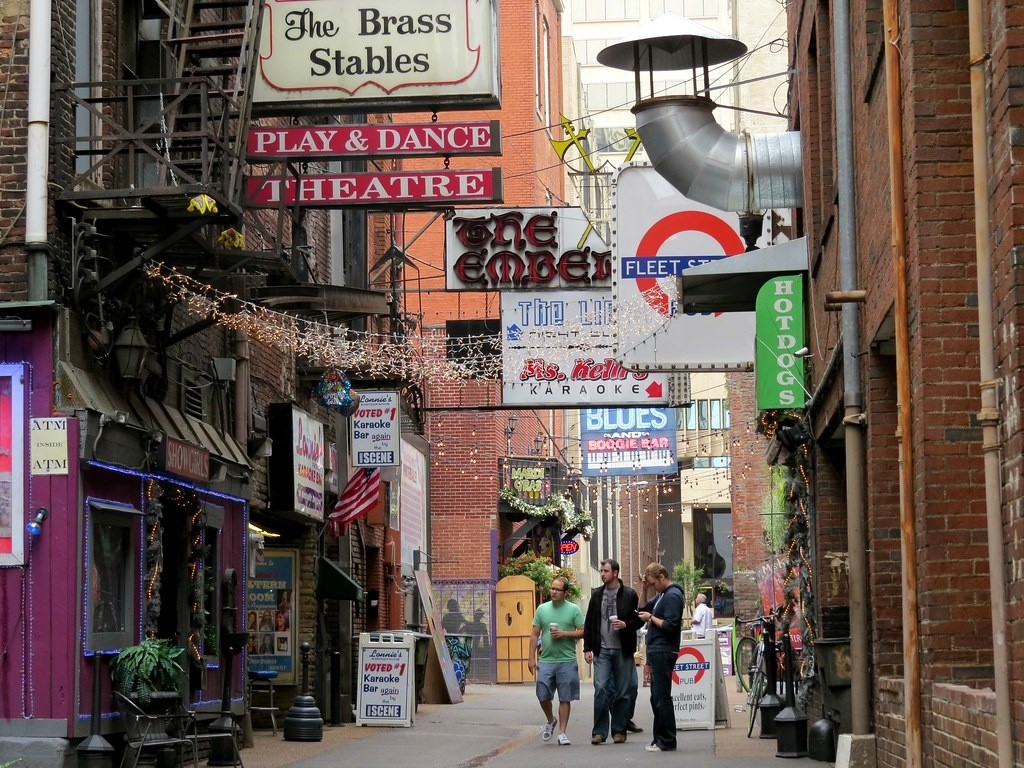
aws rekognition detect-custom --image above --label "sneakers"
[542,718,558,741]
[557,733,571,745]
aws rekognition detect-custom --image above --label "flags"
[327,466,381,536]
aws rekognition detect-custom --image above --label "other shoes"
[626,721,643,733]
[614,734,625,742]
[646,744,663,752]
[591,735,606,744]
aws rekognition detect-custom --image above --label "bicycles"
[733,610,784,738]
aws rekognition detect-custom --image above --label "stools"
[247,671,280,737]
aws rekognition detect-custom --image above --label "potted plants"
[110,638,187,714]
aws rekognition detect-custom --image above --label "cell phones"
[635,609,640,615]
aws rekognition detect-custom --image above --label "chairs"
[112,691,244,768]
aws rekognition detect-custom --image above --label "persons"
[584,559,638,744]
[528,577,585,748]
[605,578,644,734]
[635,562,685,751]
[246,609,289,654]
[691,593,712,640]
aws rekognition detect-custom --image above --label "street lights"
[634,480,650,580]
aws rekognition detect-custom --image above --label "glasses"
[551,587,564,592]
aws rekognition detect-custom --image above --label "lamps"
[116,316,150,379]
[528,434,542,456]
[26,507,49,536]
[504,412,518,436]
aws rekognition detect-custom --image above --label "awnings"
[677,235,808,314]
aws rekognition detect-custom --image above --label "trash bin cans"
[812,637,852,752]
[353,628,433,713]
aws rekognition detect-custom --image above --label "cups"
[550,622,559,630]
[609,614,618,621]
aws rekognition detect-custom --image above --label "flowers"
[498,487,595,542]
[498,550,581,602]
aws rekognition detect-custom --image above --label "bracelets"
[649,614,653,622]
